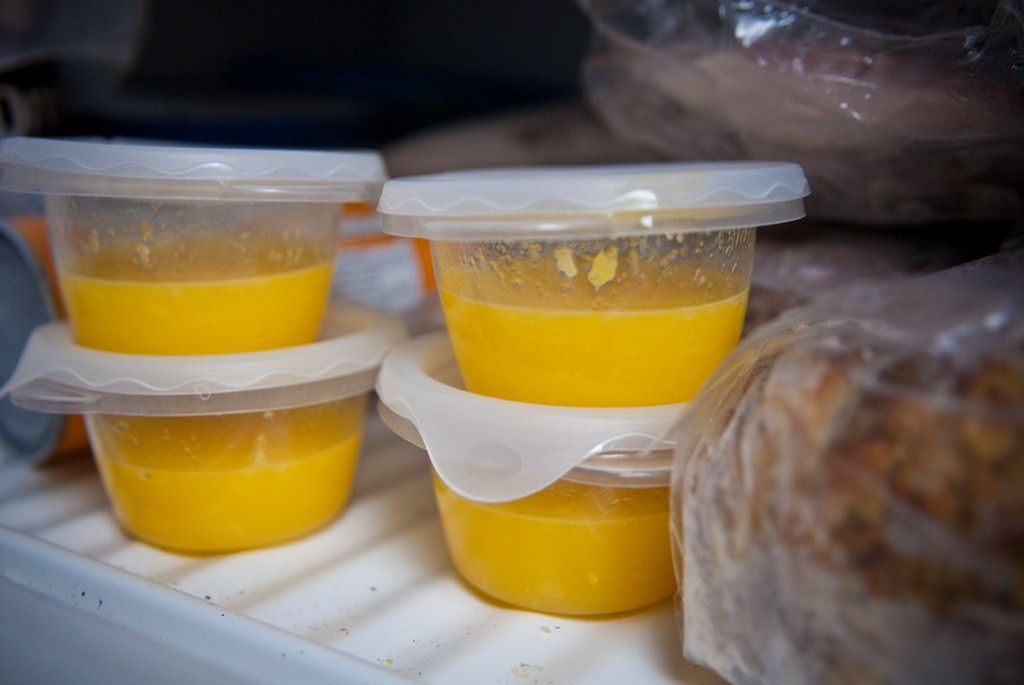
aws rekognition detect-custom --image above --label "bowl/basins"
[0,139,812,617]
